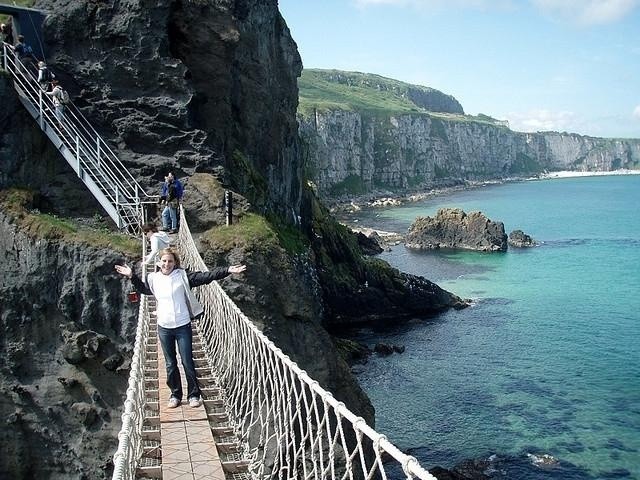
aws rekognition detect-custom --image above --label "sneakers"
[166,397,182,408]
[168,228,178,235]
[189,399,201,408]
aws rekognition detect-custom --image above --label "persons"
[158,171,183,234]
[140,222,171,272]
[5,34,33,84]
[114,247,246,408]
[37,61,55,109]
[46,80,66,132]
[0,23,8,48]
